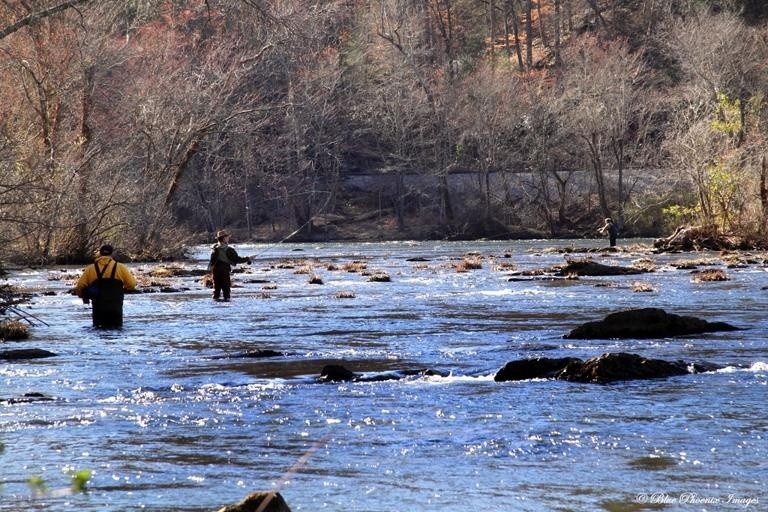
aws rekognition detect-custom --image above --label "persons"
[75,244,137,329]
[207,229,256,302]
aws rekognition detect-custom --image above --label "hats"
[214,229,232,239]
